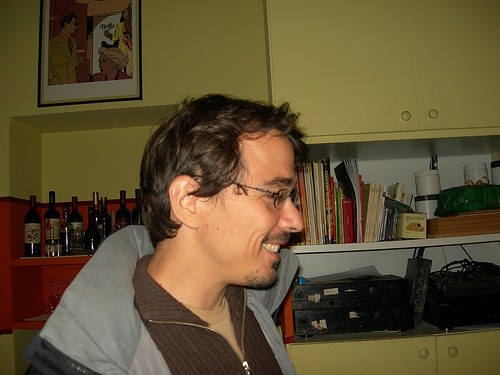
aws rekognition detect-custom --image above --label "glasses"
[185,172,300,210]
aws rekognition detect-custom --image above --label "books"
[284,154,414,247]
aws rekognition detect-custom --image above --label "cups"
[490,159,500,186]
[413,169,441,196]
[462,161,489,187]
[413,195,439,220]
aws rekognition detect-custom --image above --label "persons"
[20,95,308,375]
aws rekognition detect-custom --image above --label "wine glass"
[46,277,72,313]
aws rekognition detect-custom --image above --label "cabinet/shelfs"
[264,1,500,375]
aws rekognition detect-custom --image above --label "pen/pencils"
[430,155,438,170]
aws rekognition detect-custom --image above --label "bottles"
[26,188,145,257]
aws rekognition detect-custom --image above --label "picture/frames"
[35,0,145,112]
